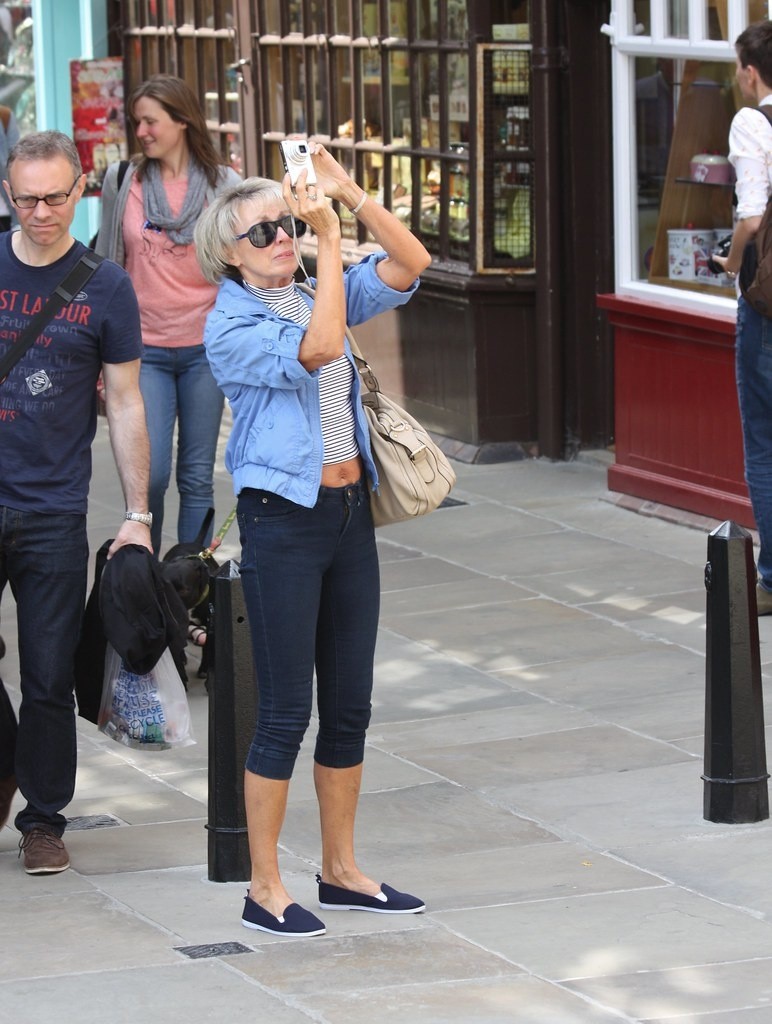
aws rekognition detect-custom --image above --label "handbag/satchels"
[97,641,197,751]
[296,282,456,527]
[733,104,772,318]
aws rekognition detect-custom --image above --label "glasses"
[233,215,307,248]
[10,175,81,209]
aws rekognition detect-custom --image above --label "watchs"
[124,511,152,528]
[725,267,738,278]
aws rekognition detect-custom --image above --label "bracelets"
[351,192,367,215]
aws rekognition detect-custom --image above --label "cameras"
[707,234,732,274]
[279,140,317,189]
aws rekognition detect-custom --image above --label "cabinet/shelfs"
[647,6,736,301]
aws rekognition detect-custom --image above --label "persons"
[692,235,724,279]
[96,76,244,559]
[0,133,154,877]
[711,25,772,615]
[0,0,21,232]
[195,138,431,936]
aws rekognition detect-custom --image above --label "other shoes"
[241,888,326,937]
[18,827,71,874]
[756,579,772,616]
[0,771,16,830]
[316,874,427,913]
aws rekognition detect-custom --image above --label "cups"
[689,150,731,183]
[715,229,733,255]
[666,228,713,282]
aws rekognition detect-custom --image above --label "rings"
[308,194,317,201]
